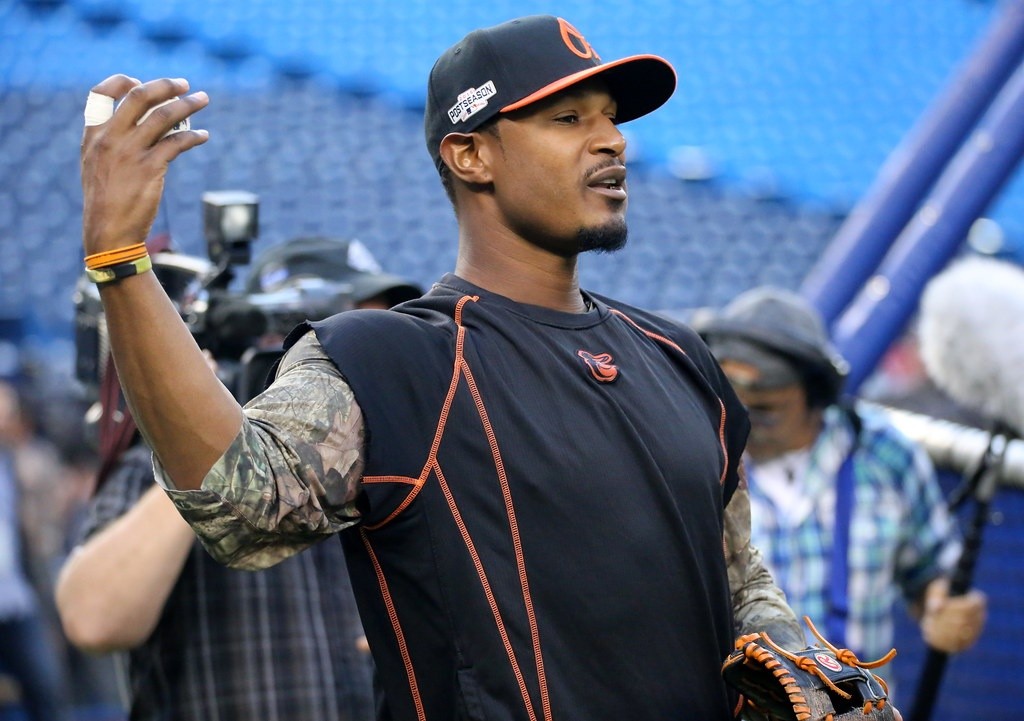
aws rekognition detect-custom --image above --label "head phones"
[690,309,850,410]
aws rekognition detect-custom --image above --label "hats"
[245,235,423,310]
[699,285,851,405]
[426,16,677,175]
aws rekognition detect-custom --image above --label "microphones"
[917,256,1024,437]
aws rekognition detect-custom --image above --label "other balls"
[114,89,193,145]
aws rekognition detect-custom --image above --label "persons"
[0,337,118,721]
[81,16,909,721]
[53,236,427,721]
[689,283,987,704]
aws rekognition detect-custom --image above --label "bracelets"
[84,255,152,284]
[83,242,148,270]
